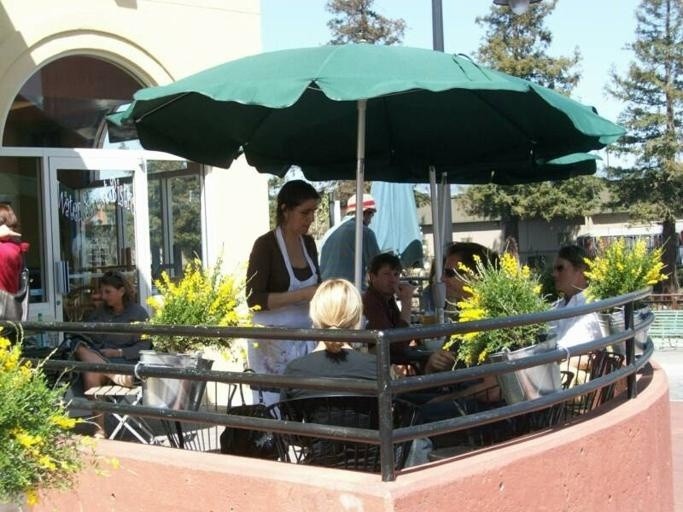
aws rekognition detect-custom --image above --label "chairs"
[263,353,623,475]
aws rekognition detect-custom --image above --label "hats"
[346,193,378,213]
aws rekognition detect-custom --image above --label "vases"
[138,350,204,411]
[599,313,655,357]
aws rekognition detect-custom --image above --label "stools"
[83,384,155,444]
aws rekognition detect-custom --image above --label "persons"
[65,270,154,439]
[655,214,683,303]
[245,181,323,420]
[0,202,31,321]
[278,191,609,439]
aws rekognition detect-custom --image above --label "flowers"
[1,338,137,511]
[443,241,556,370]
[132,253,262,364]
[571,234,666,311]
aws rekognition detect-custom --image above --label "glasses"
[444,267,465,279]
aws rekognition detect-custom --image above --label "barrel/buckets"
[595,305,648,359]
[139,351,214,411]
